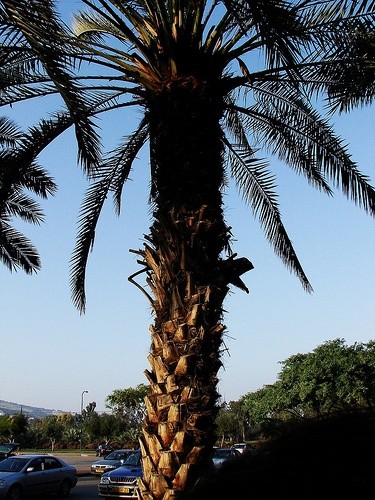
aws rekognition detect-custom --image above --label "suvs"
[98,449,142,499]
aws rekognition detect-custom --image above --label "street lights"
[75,390,89,451]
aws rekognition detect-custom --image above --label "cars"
[0,454,78,500]
[213,442,256,467]
[91,449,137,479]
[96,444,114,457]
